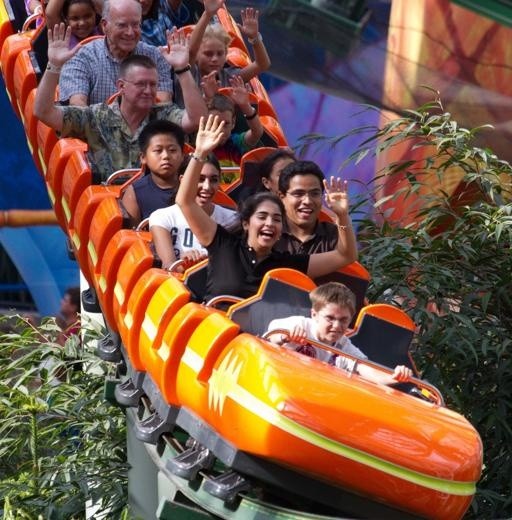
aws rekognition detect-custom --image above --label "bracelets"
[189,153,209,163]
[335,222,353,229]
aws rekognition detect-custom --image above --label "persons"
[173,113,359,316]
[148,151,238,274]
[119,120,184,232]
[24,0,270,186]
[262,281,415,390]
[24,284,84,356]
[269,161,338,259]
[259,144,298,193]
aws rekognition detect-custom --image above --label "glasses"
[123,78,161,89]
[284,190,325,200]
